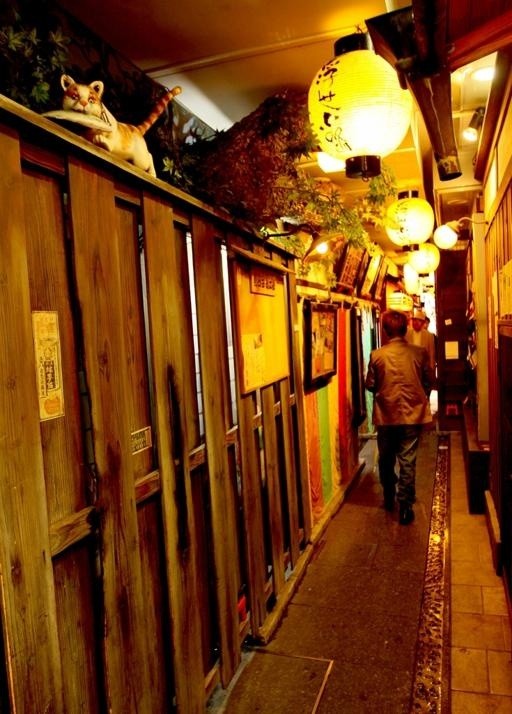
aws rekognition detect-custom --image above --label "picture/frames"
[340,240,388,299]
[306,295,340,392]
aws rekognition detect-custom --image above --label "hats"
[411,309,426,321]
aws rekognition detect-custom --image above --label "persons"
[402,310,435,370]
[421,316,430,330]
[362,307,435,526]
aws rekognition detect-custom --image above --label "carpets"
[213,644,334,713]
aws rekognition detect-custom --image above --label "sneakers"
[383,496,414,525]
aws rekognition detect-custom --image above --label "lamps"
[432,214,488,253]
[463,104,485,139]
